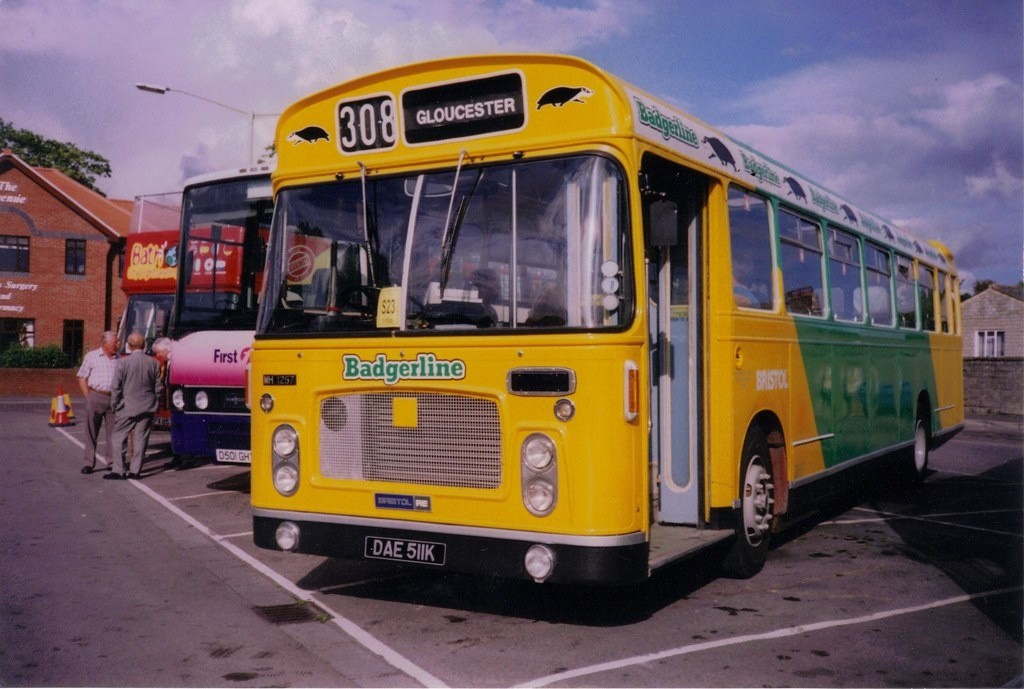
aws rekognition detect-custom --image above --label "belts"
[89,387,111,396]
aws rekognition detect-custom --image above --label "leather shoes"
[81,466,93,474]
[106,467,112,470]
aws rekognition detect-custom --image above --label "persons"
[103,332,161,480]
[77,331,121,474]
[730,253,759,308]
[462,268,501,328]
[152,337,171,362]
[524,286,568,326]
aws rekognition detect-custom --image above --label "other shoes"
[123,471,141,479]
[103,473,127,480]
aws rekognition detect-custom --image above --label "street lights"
[134,81,281,172]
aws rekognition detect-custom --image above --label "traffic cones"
[47,383,77,428]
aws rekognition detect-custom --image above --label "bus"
[244,51,968,600]
[163,160,277,469]
[112,186,187,428]
